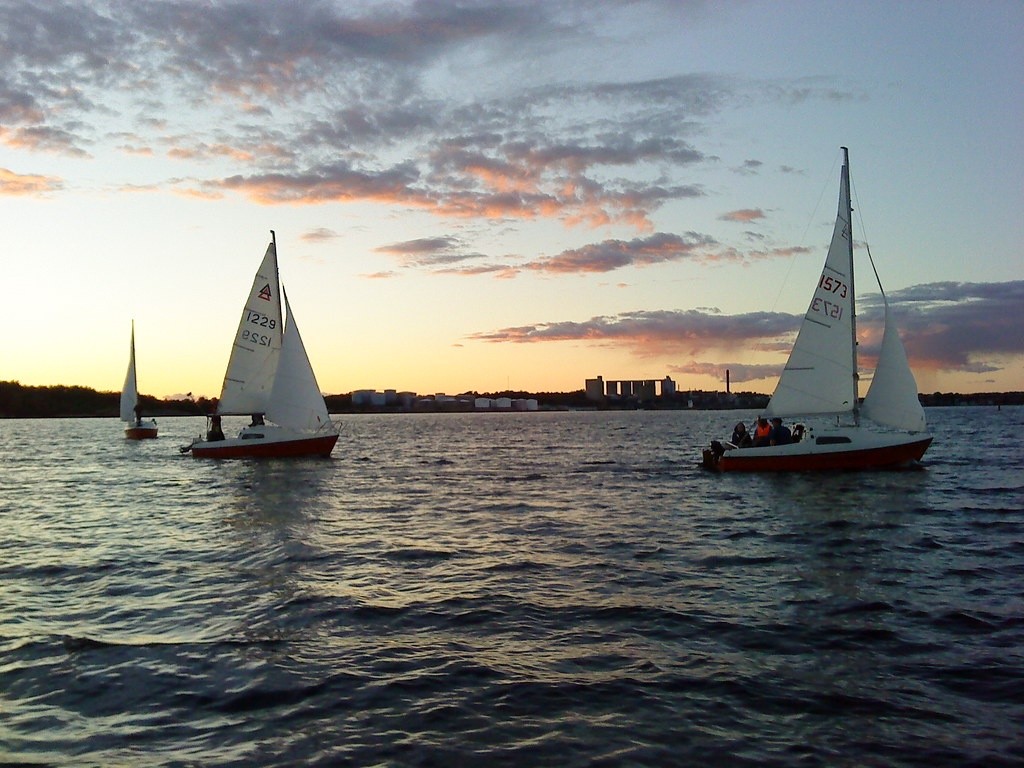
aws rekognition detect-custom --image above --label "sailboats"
[180,228,349,464]
[699,146,938,474]
[118,318,159,440]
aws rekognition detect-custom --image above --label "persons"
[732,422,752,448]
[766,417,792,446]
[752,415,772,447]
